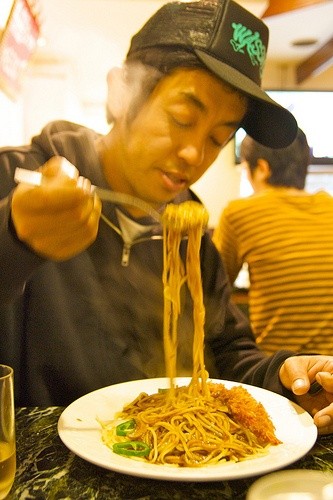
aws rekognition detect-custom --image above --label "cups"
[0,365,16,500]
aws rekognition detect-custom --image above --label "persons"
[212,127,333,354]
[0,1,333,434]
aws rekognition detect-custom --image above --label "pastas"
[95,197,285,467]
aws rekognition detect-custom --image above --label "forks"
[12,169,195,230]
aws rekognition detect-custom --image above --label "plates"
[246,469,333,500]
[57,377,318,482]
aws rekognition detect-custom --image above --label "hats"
[127,0,298,150]
